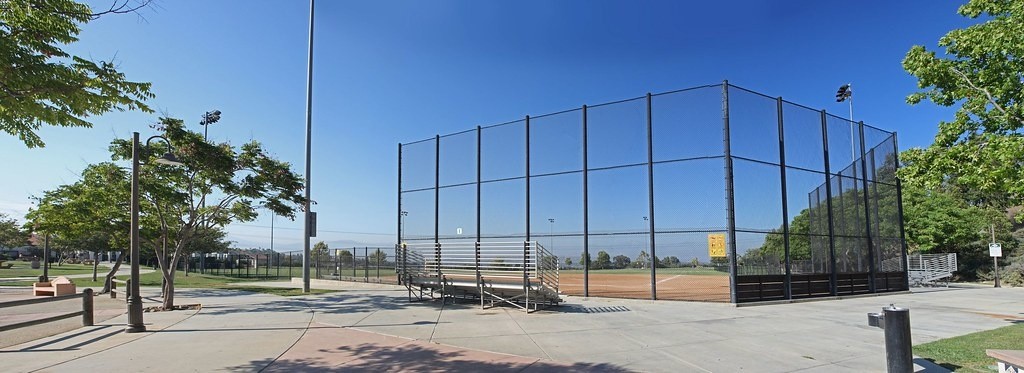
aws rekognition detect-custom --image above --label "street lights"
[642,216,648,269]
[548,218,555,273]
[198,109,221,273]
[836,82,862,272]
[124,131,185,333]
[401,210,408,245]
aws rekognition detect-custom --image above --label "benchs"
[32,274,76,296]
[409,261,559,313]
[909,265,953,291]
[988,348,1024,373]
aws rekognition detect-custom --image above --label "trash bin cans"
[867,303,914,373]
